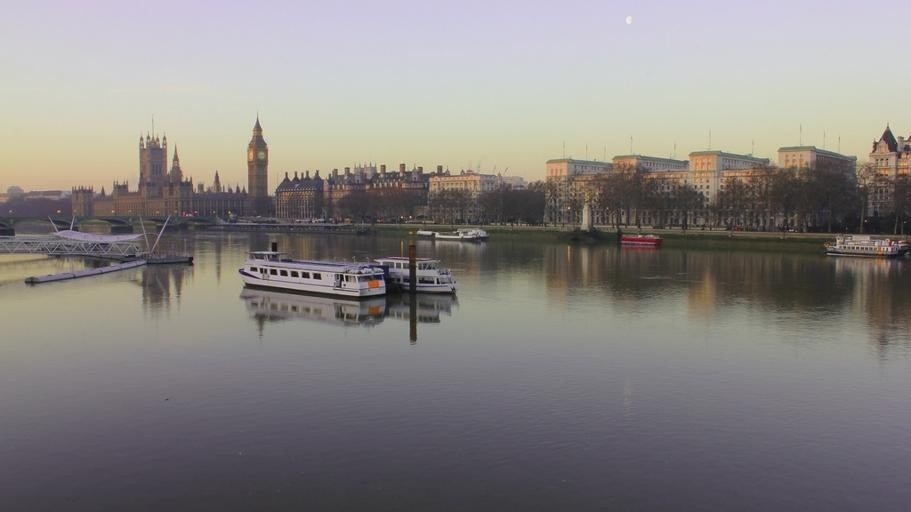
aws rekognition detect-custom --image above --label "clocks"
[257,151,266,160]
[248,151,254,161]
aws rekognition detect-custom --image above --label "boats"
[620,234,663,244]
[824,233,911,256]
[417,228,490,239]
[238,239,455,296]
